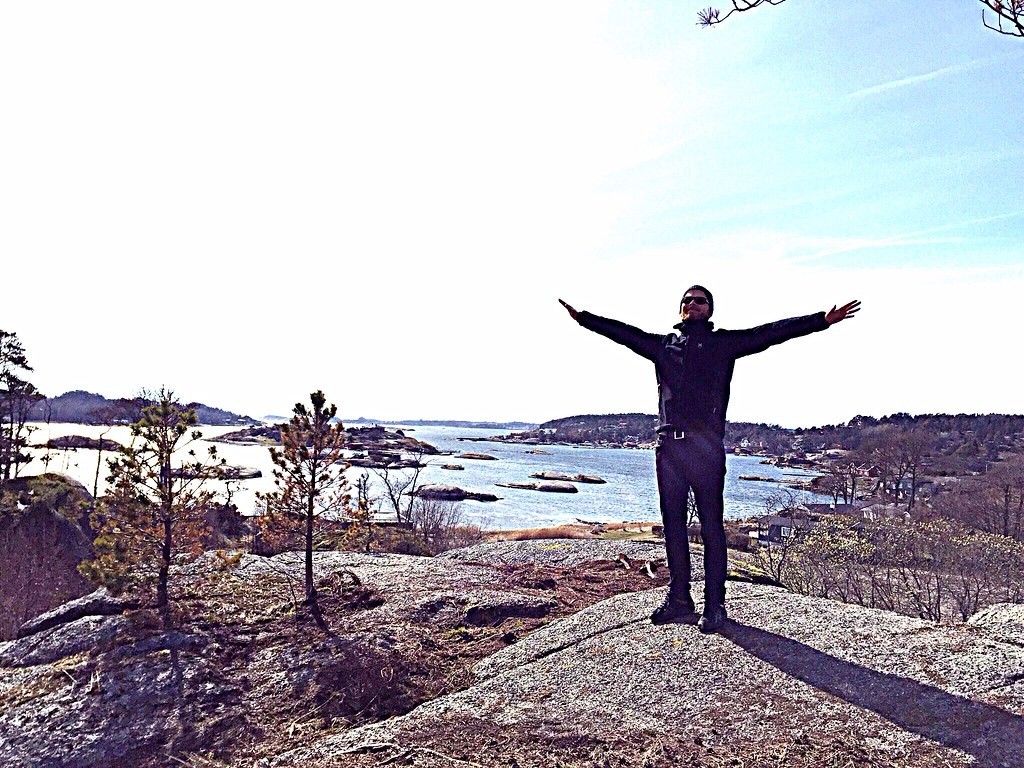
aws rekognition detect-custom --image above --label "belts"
[659,430,718,439]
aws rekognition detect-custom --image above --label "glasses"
[682,296,709,305]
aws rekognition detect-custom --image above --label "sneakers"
[698,603,727,631]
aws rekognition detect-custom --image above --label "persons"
[557,284,862,632]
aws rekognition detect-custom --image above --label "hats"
[679,285,713,318]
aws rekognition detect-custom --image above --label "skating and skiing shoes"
[649,591,695,620]
